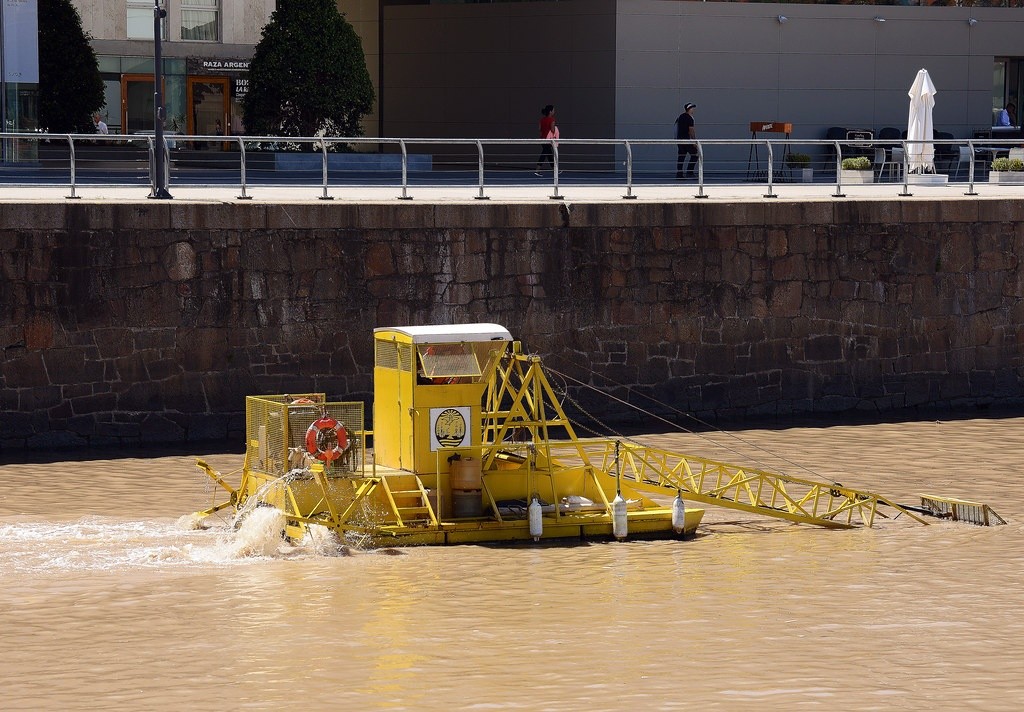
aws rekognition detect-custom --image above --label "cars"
[128,130,187,150]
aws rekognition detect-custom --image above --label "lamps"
[969,18,977,28]
[871,16,885,23]
[777,14,789,24]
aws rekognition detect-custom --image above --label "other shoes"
[686,172,697,179]
[676,172,685,179]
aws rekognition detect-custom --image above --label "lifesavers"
[305,419,347,461]
[426,345,466,384]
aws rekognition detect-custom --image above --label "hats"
[684,103,696,112]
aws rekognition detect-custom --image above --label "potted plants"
[989,157,1024,182]
[786,152,814,182]
[841,156,874,184]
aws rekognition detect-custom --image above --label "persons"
[535,105,563,177]
[93,113,108,134]
[994,103,1015,126]
[677,102,700,177]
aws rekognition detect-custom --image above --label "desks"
[974,147,1010,171]
[746,122,792,182]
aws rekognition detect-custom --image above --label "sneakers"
[556,170,562,175]
[535,172,544,177]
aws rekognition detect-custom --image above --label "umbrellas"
[906,68,938,174]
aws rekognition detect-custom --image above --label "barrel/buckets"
[419,489,444,519]
[449,457,481,490]
[452,490,483,517]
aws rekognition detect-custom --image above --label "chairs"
[955,146,985,180]
[822,127,954,182]
